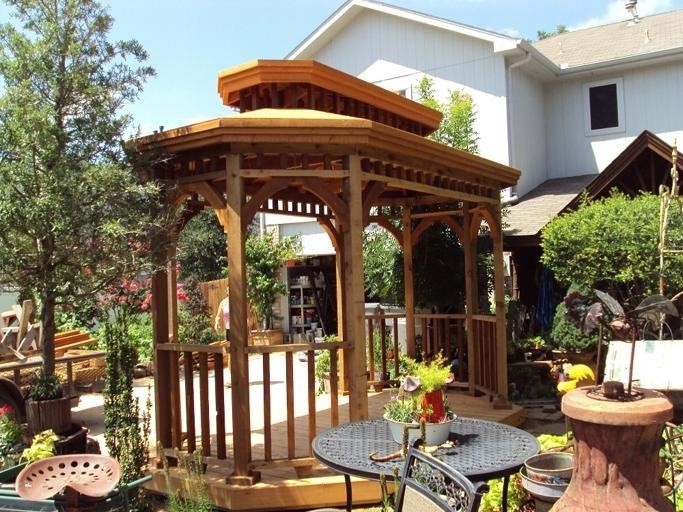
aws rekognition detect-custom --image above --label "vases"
[382,411,457,447]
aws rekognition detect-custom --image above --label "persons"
[215,286,250,388]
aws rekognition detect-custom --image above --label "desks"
[311,415,542,512]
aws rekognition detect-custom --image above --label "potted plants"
[22,364,73,435]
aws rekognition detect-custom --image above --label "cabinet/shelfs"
[289,283,327,334]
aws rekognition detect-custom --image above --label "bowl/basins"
[524,452,575,482]
[519,466,570,499]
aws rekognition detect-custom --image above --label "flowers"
[381,347,457,422]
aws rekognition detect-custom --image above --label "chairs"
[392,436,491,512]
